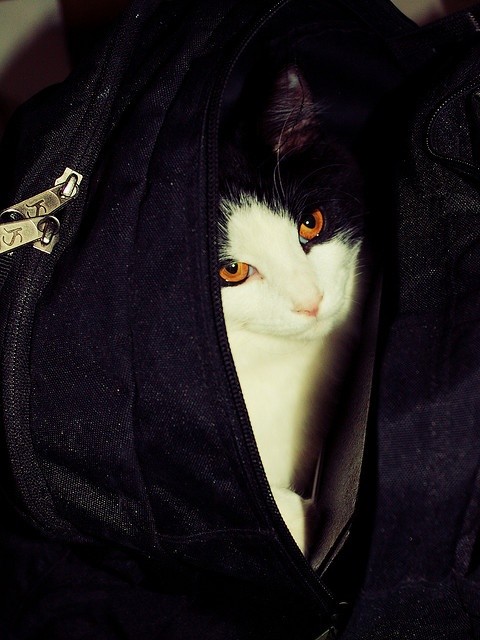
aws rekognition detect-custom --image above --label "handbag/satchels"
[0,0,480,640]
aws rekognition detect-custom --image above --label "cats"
[213,167,370,557]
